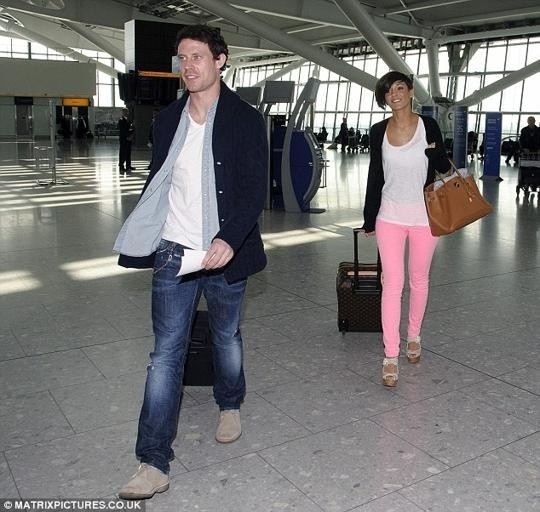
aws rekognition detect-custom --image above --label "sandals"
[381,356,398,387]
[406,336,422,363]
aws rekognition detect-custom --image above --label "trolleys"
[516,152,540,197]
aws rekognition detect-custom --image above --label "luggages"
[334,227,384,337]
[178,308,220,386]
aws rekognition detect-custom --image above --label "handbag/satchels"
[423,158,495,238]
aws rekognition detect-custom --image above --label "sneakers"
[117,461,171,500]
[216,408,242,443]
[119,164,136,172]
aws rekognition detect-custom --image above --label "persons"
[317,126,328,143]
[75,115,87,130]
[143,108,163,171]
[334,127,370,154]
[60,114,70,139]
[518,116,540,192]
[504,138,516,163]
[117,106,137,173]
[340,117,349,153]
[112,25,270,502]
[359,72,453,388]
